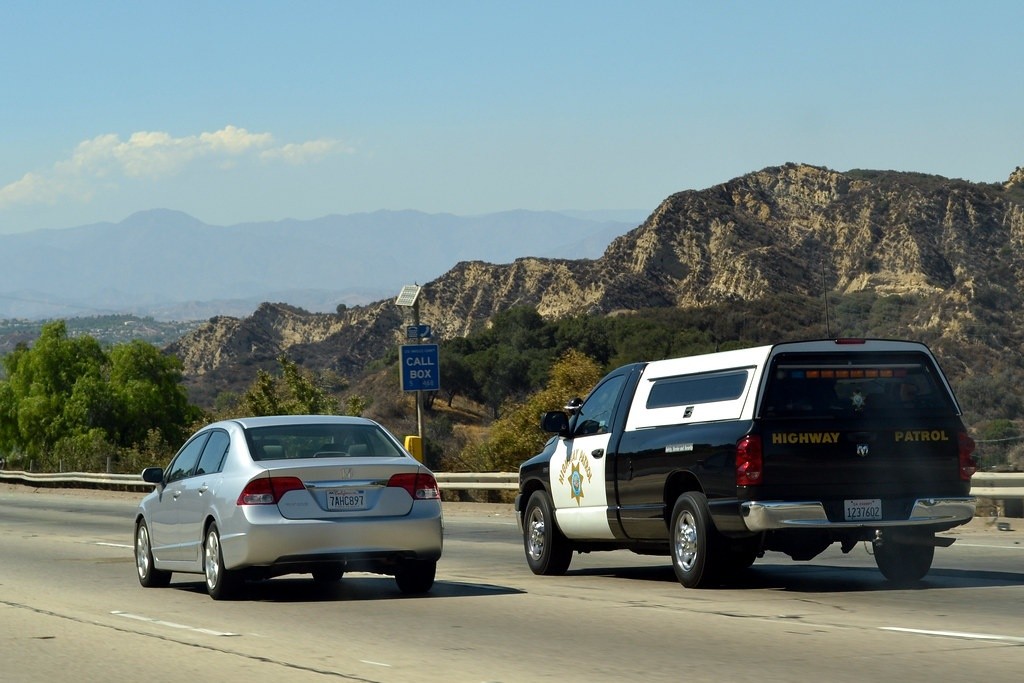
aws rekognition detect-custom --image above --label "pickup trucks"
[515,337,977,589]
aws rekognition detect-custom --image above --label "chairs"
[252,440,369,458]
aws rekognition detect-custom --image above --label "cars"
[133,414,445,600]
